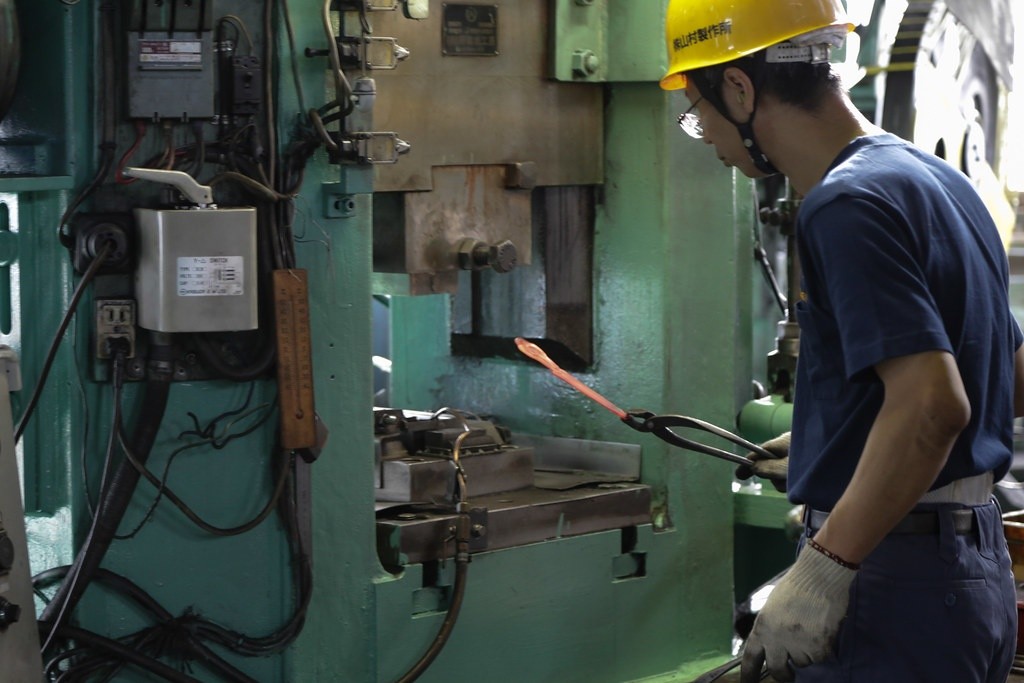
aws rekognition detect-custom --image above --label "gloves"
[740,540,862,683]
[736,429,792,493]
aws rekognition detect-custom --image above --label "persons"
[659,0,1024,682]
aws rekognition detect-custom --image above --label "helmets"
[659,0,856,91]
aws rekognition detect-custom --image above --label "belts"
[801,505,977,535]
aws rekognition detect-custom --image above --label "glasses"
[675,84,715,139]
